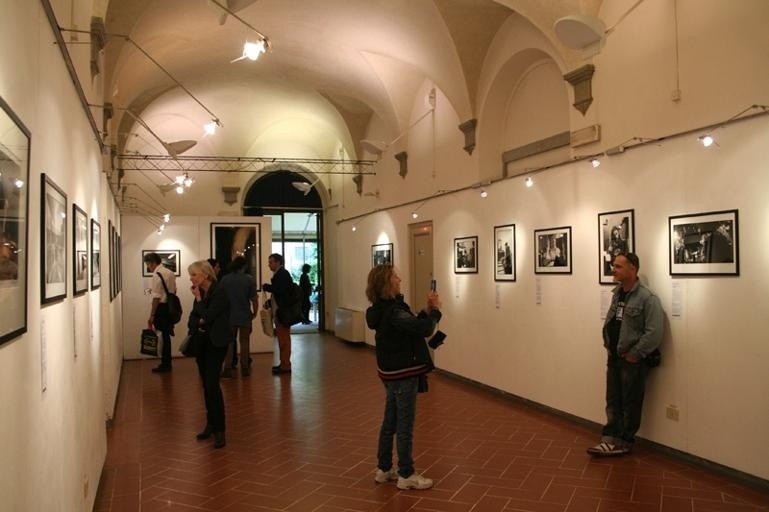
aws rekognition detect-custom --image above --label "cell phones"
[431,279,436,290]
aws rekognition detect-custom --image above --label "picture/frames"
[72,203,90,297]
[209,222,263,292]
[534,225,573,274]
[142,249,181,279]
[108,217,123,304]
[371,241,395,270]
[39,172,70,306]
[493,223,518,282]
[598,208,636,285]
[668,209,740,276]
[453,236,478,273]
[0,95,33,345]
[90,216,102,289]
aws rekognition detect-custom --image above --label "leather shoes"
[197,425,225,449]
[152,365,171,373]
[272,366,291,373]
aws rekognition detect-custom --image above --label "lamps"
[216,0,274,66]
[523,167,549,187]
[587,136,642,168]
[477,185,488,198]
[59,26,222,136]
[695,104,765,148]
[411,189,444,220]
[359,108,433,157]
[551,1,675,60]
[83,103,192,235]
[292,159,342,197]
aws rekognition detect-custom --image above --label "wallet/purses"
[428,330,446,349]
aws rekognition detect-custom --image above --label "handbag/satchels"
[166,293,182,323]
[141,329,157,356]
[179,331,201,358]
[261,308,273,337]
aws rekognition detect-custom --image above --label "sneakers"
[375,468,398,483]
[397,474,433,489]
[586,442,625,456]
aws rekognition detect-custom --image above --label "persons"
[587,252,663,456]
[298,264,315,324]
[539,234,567,267]
[501,241,510,262]
[609,226,620,238]
[186,259,228,448]
[456,244,467,268]
[468,241,475,268]
[364,264,442,490]
[205,253,302,377]
[143,252,178,375]
[604,217,630,272]
[672,222,734,265]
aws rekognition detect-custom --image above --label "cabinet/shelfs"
[334,305,366,346]
